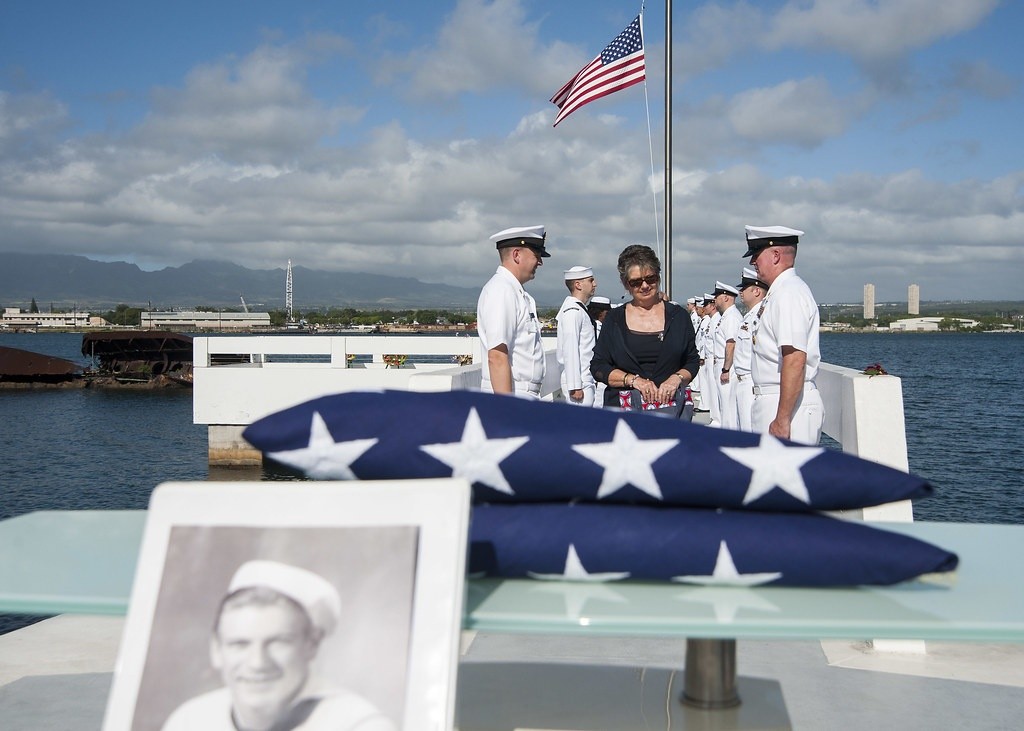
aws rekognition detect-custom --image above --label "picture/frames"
[102,477,471,731]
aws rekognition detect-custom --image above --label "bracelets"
[629,374,639,390]
[622,372,631,389]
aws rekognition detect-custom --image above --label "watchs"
[722,368,730,373]
[673,373,685,383]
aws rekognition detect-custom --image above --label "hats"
[694,296,705,307]
[701,291,717,305]
[736,266,769,289]
[490,224,551,258]
[685,296,696,306]
[228,558,342,639]
[562,264,593,281]
[712,279,739,297]
[589,295,611,307]
[741,219,804,255]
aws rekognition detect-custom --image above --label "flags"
[547,13,645,128]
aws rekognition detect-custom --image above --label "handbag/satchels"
[619,386,695,425]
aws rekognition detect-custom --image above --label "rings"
[667,393,670,395]
[645,390,650,394]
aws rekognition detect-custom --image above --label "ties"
[577,301,598,345]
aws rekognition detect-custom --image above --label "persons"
[476,224,552,402]
[161,558,393,731]
[555,265,612,409]
[742,224,826,447]
[687,266,768,434]
[591,246,700,423]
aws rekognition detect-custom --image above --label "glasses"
[625,273,662,287]
